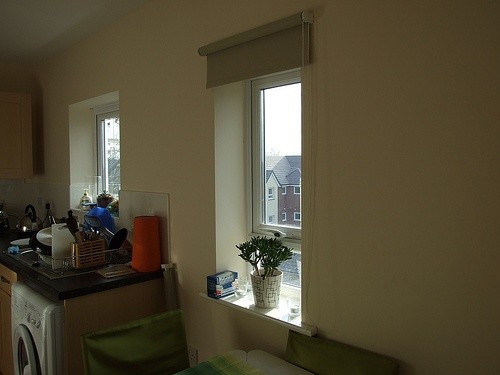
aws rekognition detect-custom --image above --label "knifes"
[66,210,83,243]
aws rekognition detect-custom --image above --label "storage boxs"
[207,270,238,299]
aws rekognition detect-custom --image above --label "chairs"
[284,328,400,375]
[80,309,191,375]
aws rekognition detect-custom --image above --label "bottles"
[0,202,11,232]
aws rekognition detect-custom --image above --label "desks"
[174,348,318,375]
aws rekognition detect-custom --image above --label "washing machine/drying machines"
[10,280,65,375]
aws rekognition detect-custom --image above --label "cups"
[231,280,249,296]
[286,298,301,316]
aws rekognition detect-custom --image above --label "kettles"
[17,204,42,237]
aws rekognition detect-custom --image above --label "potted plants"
[96,190,113,207]
[235,235,297,309]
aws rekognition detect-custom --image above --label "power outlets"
[42,199,52,211]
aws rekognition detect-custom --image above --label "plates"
[37,227,53,246]
[10,238,29,248]
